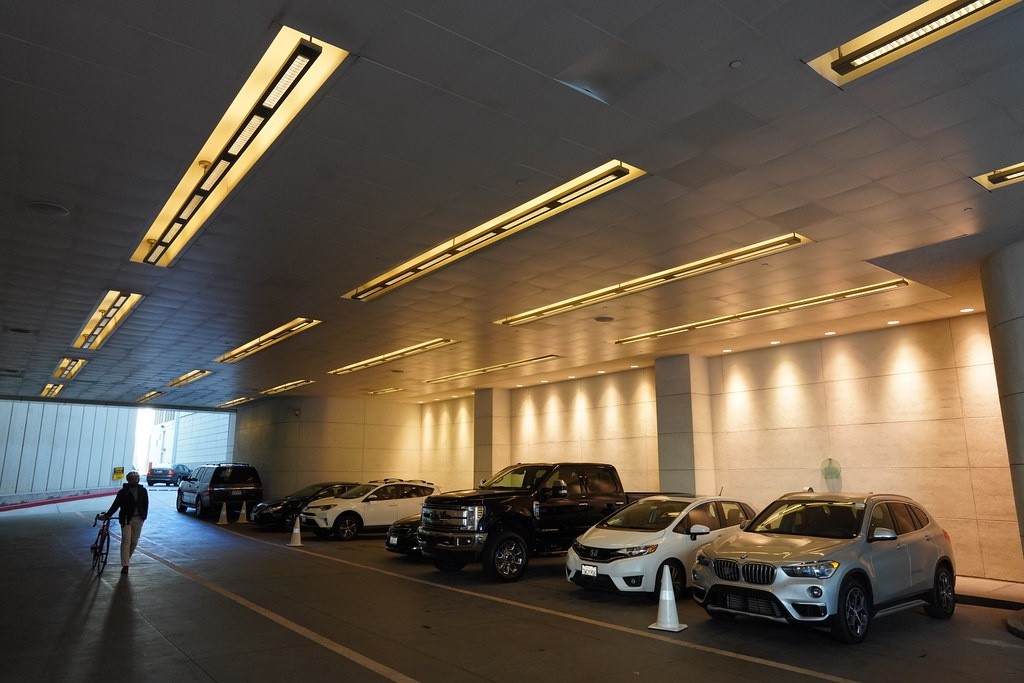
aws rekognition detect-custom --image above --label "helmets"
[126,471,139,480]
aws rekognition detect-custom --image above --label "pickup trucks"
[419,462,695,584]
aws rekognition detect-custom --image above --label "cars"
[147,463,193,487]
[385,511,423,557]
[565,495,761,606]
[250,482,359,533]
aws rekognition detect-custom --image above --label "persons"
[99,472,149,575]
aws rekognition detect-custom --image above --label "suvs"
[692,487,957,647]
[300,478,442,541]
[176,462,264,519]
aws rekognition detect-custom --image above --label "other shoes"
[121,565,128,574]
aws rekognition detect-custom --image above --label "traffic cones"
[216,502,228,524]
[235,501,248,523]
[286,516,304,546]
[648,565,688,632]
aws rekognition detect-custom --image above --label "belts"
[133,515,140,517]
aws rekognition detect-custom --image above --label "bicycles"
[90,511,119,575]
